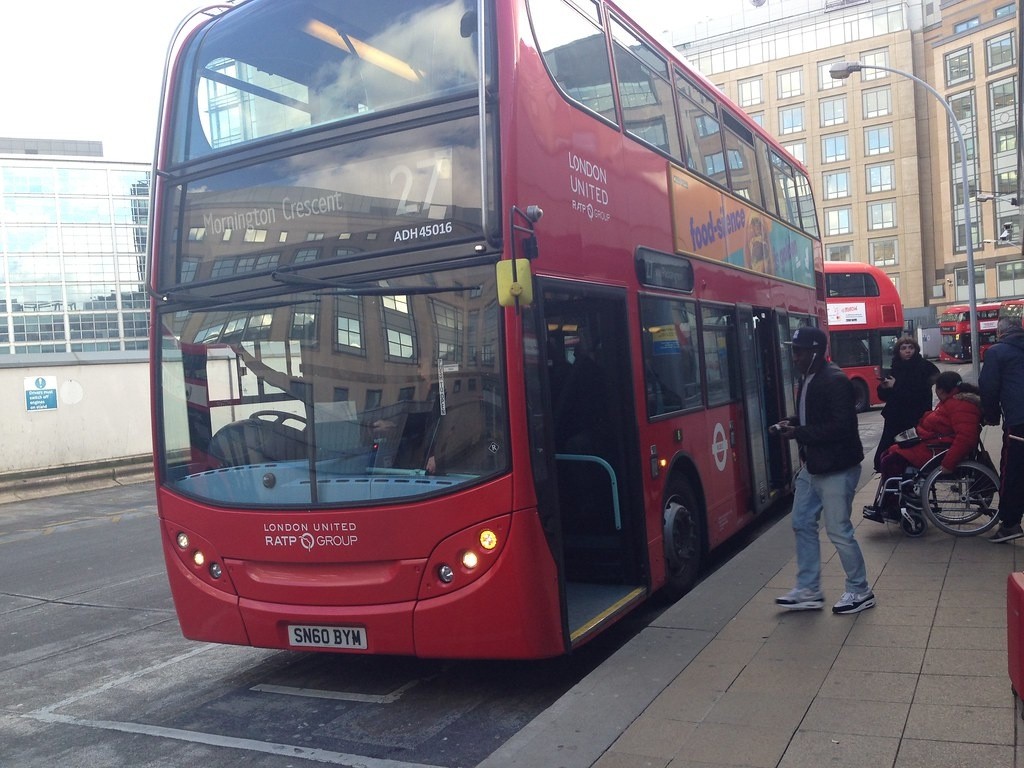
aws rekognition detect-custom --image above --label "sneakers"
[832,587,876,613]
[775,588,825,608]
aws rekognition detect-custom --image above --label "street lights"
[829,62,980,391]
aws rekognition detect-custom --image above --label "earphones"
[812,353,816,360]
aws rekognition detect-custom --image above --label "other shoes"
[864,510,901,523]
[863,505,875,512]
[988,522,1024,543]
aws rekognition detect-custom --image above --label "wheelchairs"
[863,421,1001,538]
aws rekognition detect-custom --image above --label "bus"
[940,301,1001,363]
[822,261,904,413]
[999,300,1024,328]
[143,0,831,660]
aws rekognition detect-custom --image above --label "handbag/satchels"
[894,427,920,449]
[969,438,999,499]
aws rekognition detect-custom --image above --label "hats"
[781,327,828,350]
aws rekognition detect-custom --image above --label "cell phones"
[877,377,885,382]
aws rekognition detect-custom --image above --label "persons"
[873,335,941,475]
[862,370,981,525]
[773,327,875,615]
[373,343,506,475]
[313,53,374,124]
[230,338,368,456]
[977,314,1024,542]
